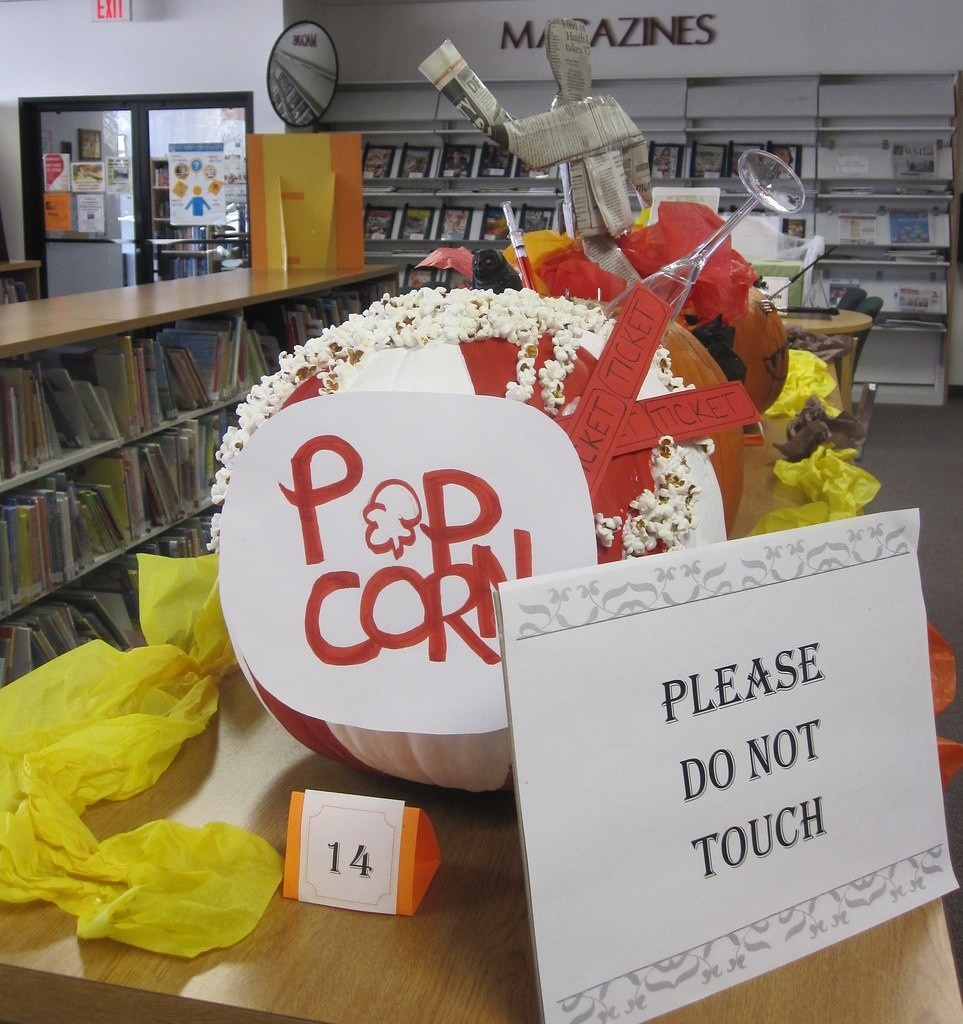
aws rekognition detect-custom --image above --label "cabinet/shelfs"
[1,71,962,689]
[269,59,325,126]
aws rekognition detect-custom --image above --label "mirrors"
[267,22,339,127]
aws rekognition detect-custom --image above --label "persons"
[772,146,793,173]
[659,147,671,177]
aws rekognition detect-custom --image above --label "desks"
[1,697,960,1022]
[777,306,872,412]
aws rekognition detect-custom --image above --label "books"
[153,167,208,279]
[884,250,945,262]
[0,277,397,688]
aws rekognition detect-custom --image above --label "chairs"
[841,289,869,312]
[838,296,883,384]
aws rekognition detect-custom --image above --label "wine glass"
[602,149,805,345]
